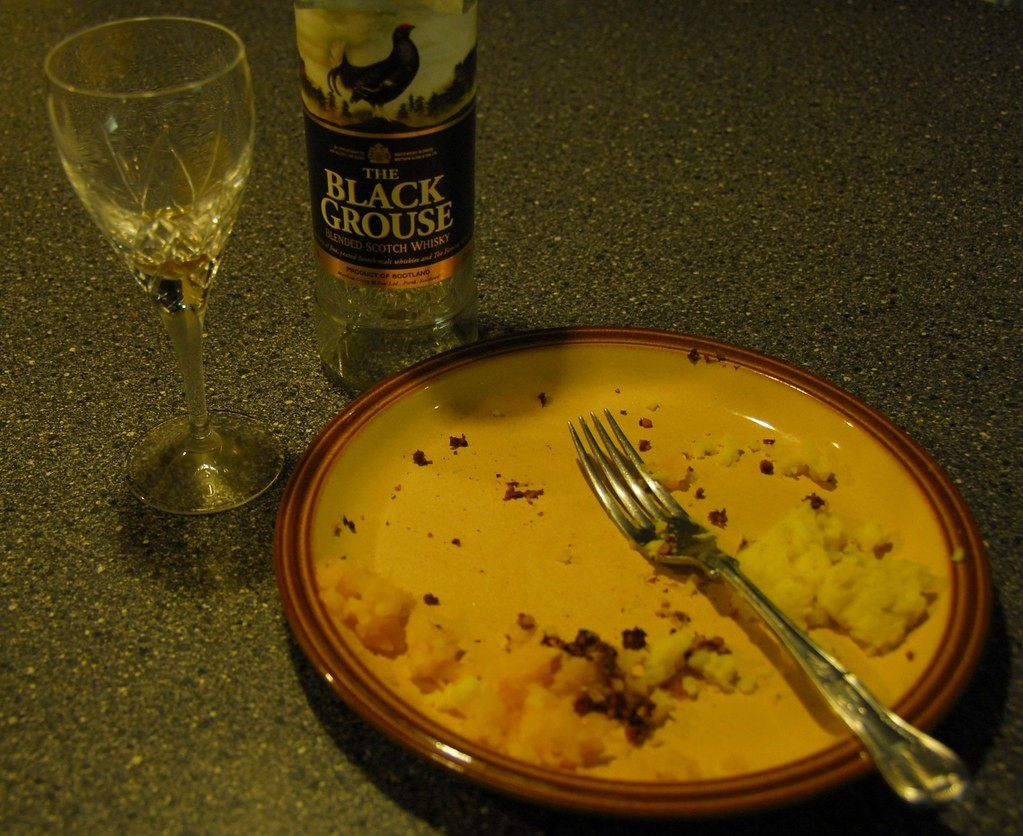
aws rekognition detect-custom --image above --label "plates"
[272,326,988,818]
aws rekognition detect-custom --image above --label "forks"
[566,408,971,805]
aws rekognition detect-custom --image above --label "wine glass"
[43,16,283,515]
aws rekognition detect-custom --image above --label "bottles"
[292,0,477,396]
